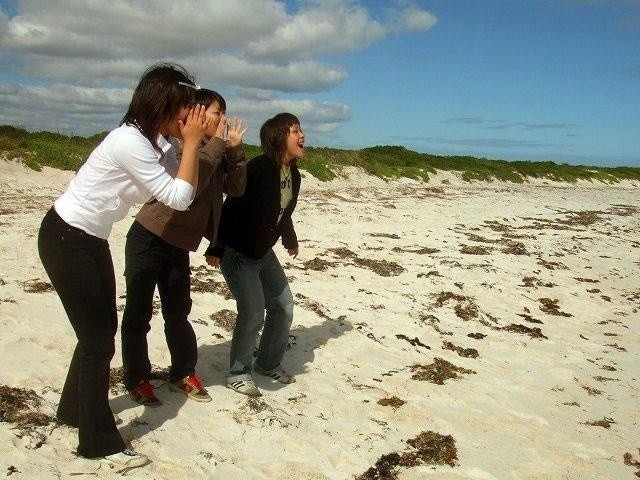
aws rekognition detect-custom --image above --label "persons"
[36,60,213,469]
[202,112,307,396]
[119,87,250,409]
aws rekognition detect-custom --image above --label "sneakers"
[225,371,261,398]
[124,375,161,407]
[57,411,149,468]
[253,358,296,384]
[169,372,212,402]
